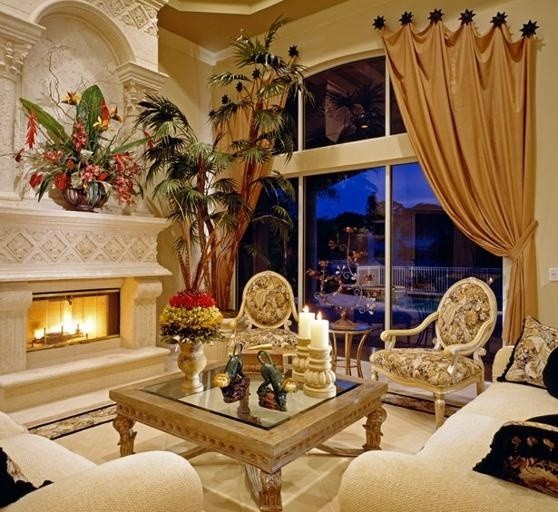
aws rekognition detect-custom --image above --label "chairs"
[223,270,301,368]
[370,276,500,435]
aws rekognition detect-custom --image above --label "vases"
[176,340,208,394]
[63,185,110,212]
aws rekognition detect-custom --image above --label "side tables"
[330,322,385,379]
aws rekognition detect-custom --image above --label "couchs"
[343,331,558,511]
[0,408,204,512]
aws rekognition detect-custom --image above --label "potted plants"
[132,11,309,363]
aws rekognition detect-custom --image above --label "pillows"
[472,412,558,496]
[502,315,558,389]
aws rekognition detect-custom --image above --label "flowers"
[14,71,147,210]
[160,193,234,344]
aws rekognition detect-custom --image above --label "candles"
[298,304,314,339]
[311,311,331,346]
